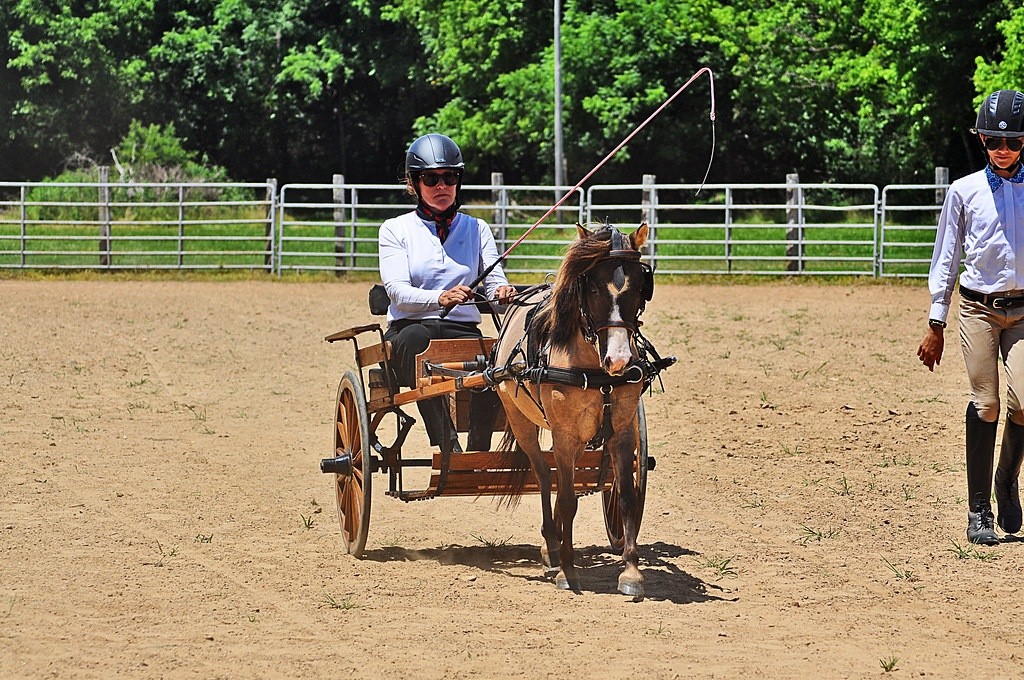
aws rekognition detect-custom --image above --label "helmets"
[976,90,1024,137]
[406,134,465,197]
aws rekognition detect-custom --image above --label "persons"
[378,133,521,475]
[916,90,1024,544]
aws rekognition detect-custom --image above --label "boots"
[994,413,1024,533]
[966,401,999,544]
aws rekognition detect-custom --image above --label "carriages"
[319,212,679,601]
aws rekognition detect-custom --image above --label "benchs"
[327,286,617,497]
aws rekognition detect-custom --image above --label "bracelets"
[929,319,946,329]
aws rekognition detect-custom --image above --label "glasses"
[416,171,458,187]
[985,137,1024,151]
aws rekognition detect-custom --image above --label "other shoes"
[439,439,472,473]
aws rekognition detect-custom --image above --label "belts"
[959,285,1024,309]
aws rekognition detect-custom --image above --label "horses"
[494,221,650,597]
